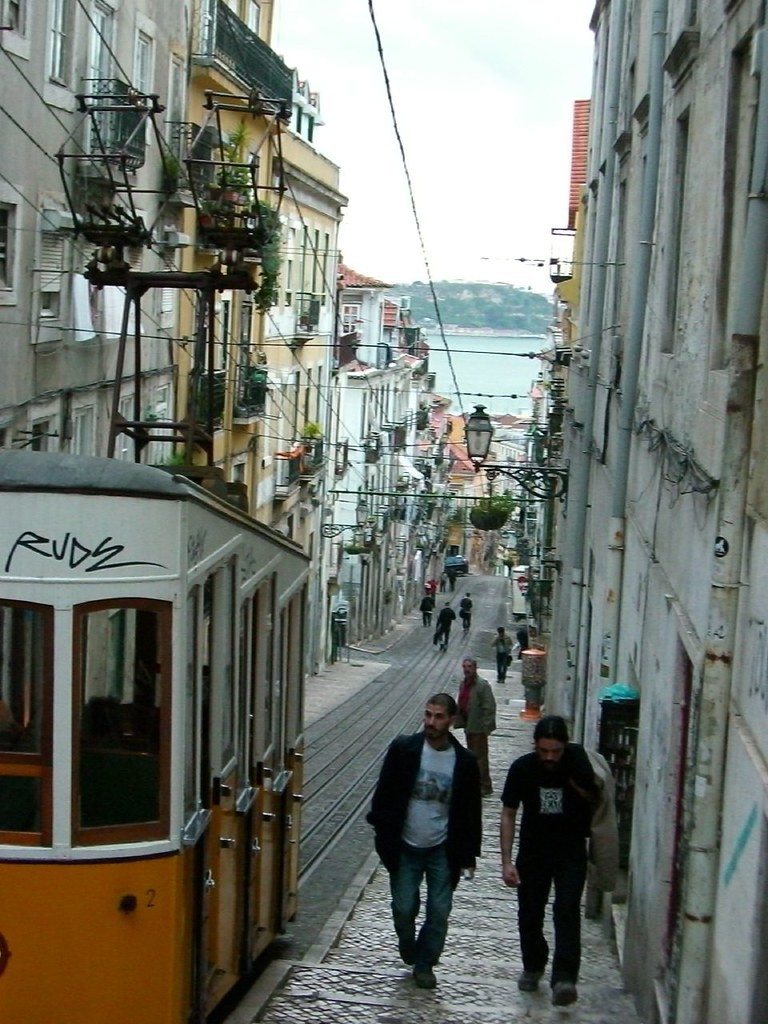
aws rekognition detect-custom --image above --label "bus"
[1,449,311,1024]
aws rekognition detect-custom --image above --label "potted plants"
[200,120,253,228]
[299,311,308,325]
[468,489,518,531]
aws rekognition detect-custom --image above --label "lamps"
[321,499,369,538]
[461,404,568,502]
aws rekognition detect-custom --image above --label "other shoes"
[551,980,577,1006]
[400,933,416,965]
[498,676,505,683]
[444,647,447,651]
[480,784,493,796]
[414,961,437,988]
[518,963,545,990]
[440,645,444,650]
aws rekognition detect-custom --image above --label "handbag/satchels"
[507,656,512,665]
[433,633,438,645]
[460,608,465,618]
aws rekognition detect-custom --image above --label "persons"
[449,568,457,591]
[459,592,473,632]
[439,572,447,592]
[420,591,435,627]
[366,691,483,989]
[491,626,512,685]
[456,657,497,797]
[433,601,456,653]
[500,714,604,1007]
